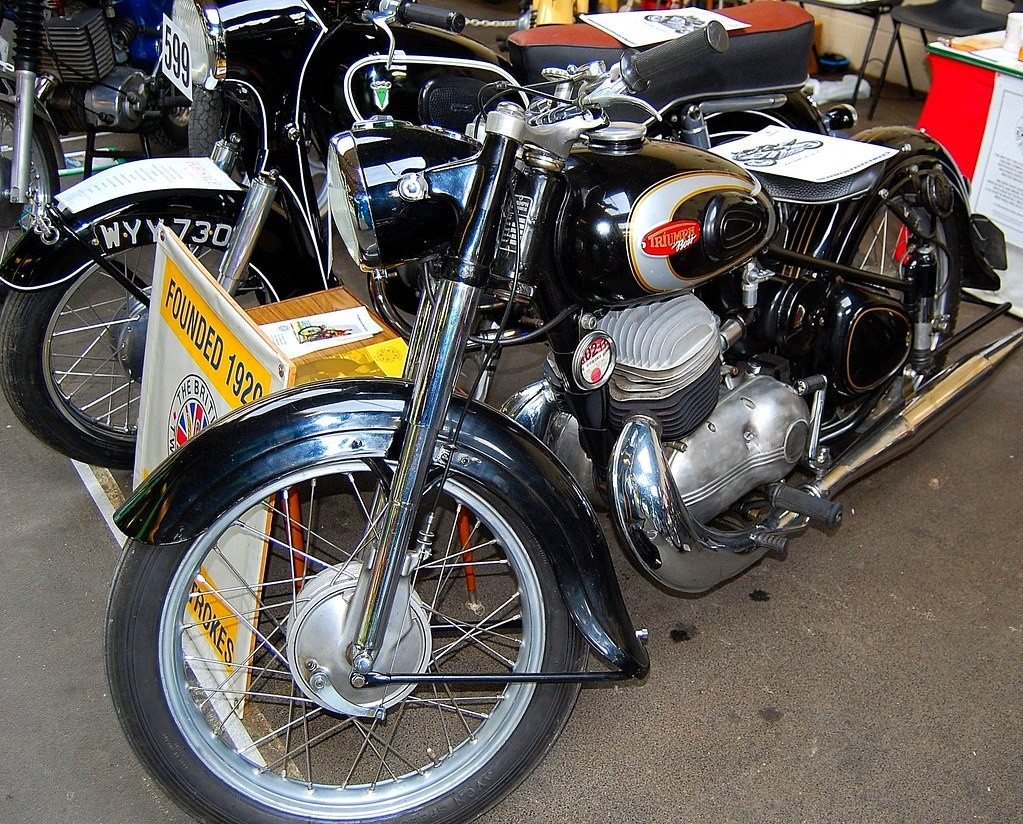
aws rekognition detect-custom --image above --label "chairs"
[784,0,918,108]
[868,0,1023,121]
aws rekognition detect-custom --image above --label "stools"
[245,286,485,618]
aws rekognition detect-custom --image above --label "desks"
[894,30,1023,320]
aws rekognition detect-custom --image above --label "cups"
[1002,12,1023,53]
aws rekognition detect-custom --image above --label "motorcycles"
[1,0,1023,824]
